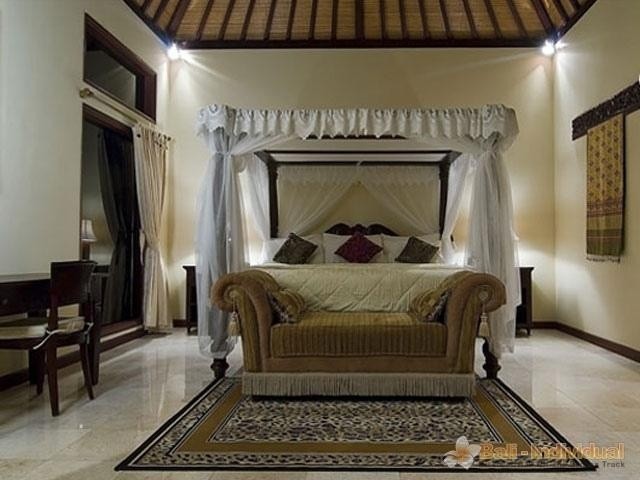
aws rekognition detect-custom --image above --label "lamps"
[81,219,98,263]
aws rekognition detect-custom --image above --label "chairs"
[0,259,97,417]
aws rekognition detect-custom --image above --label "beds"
[210,222,502,379]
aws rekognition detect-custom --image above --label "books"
[0,308,31,327]
[30,295,80,318]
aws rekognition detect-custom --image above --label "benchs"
[209,268,507,403]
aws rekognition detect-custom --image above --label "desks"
[1,272,111,397]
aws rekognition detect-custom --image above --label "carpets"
[113,375,594,473]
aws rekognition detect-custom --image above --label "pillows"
[262,232,442,265]
[264,285,306,324]
[408,288,449,324]
